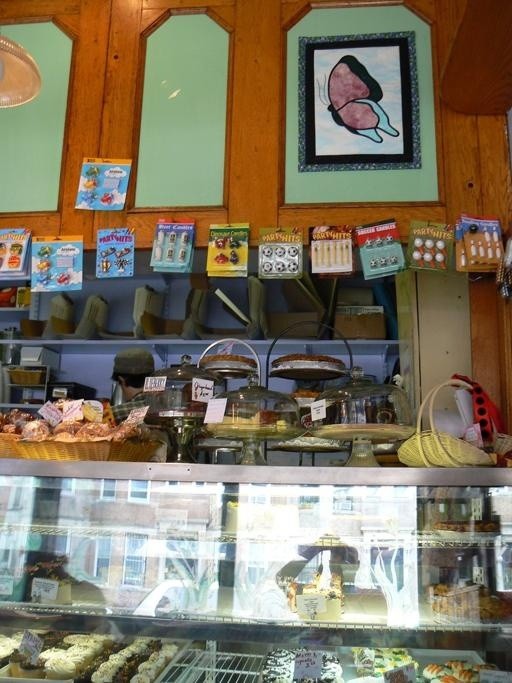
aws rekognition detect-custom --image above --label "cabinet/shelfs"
[0,0,449,251]
[0,457,512,682]
[0,306,401,412]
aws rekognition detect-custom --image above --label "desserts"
[1,629,117,680]
[129,644,177,683]
[91,638,162,683]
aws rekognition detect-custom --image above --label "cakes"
[299,508,362,537]
[157,502,210,528]
[350,645,420,683]
[263,647,344,683]
[425,577,508,622]
[223,502,299,536]
[429,519,501,546]
[29,574,74,605]
[87,502,154,528]
[286,569,345,617]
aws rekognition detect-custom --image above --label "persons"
[276,532,395,627]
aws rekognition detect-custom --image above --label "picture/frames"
[297,30,421,173]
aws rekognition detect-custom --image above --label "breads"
[424,660,497,683]
[0,398,156,440]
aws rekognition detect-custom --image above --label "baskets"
[1,431,162,460]
[397,379,512,466]
